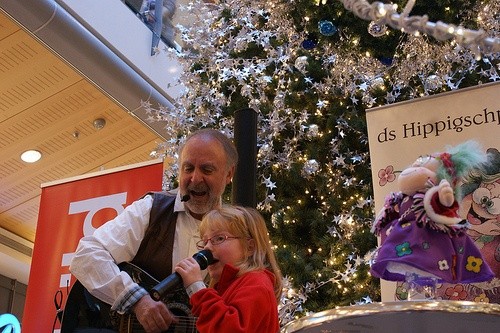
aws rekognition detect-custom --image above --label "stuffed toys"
[371,139,492,299]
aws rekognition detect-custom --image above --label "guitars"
[60,261,198,333]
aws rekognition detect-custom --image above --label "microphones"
[54,299,63,323]
[181,195,191,202]
[149,250,213,302]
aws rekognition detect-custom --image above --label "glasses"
[196,234,249,250]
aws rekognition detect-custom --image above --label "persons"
[174,204,282,333]
[69,128,237,333]
